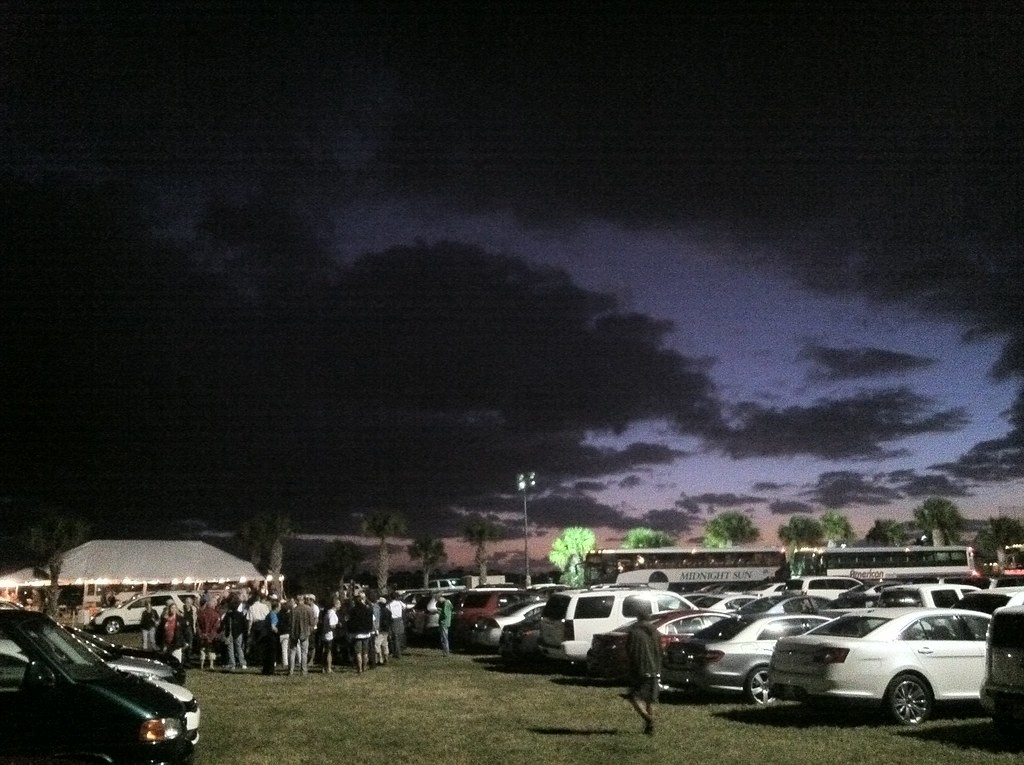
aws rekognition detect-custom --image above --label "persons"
[160,598,178,630]
[431,591,455,658]
[160,603,190,665]
[181,596,197,654]
[194,585,420,675]
[108,589,117,609]
[618,605,664,737]
[138,600,158,653]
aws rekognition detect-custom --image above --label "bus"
[996,543,1024,579]
[789,544,976,584]
[571,544,790,590]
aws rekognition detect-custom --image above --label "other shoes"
[644,718,654,734]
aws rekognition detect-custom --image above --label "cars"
[379,578,1024,733]
[337,583,363,600]
[0,597,201,765]
[94,589,201,636]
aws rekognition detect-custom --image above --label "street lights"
[518,469,536,590]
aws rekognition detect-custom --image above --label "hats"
[376,597,386,604]
[304,594,315,601]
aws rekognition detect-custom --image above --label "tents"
[0,536,285,621]
